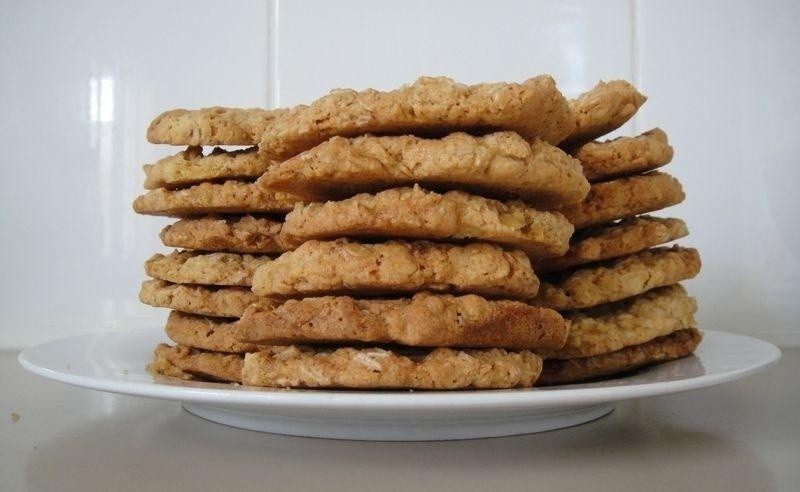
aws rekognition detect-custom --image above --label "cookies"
[132,75,701,388]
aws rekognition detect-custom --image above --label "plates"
[16,328,785,445]
